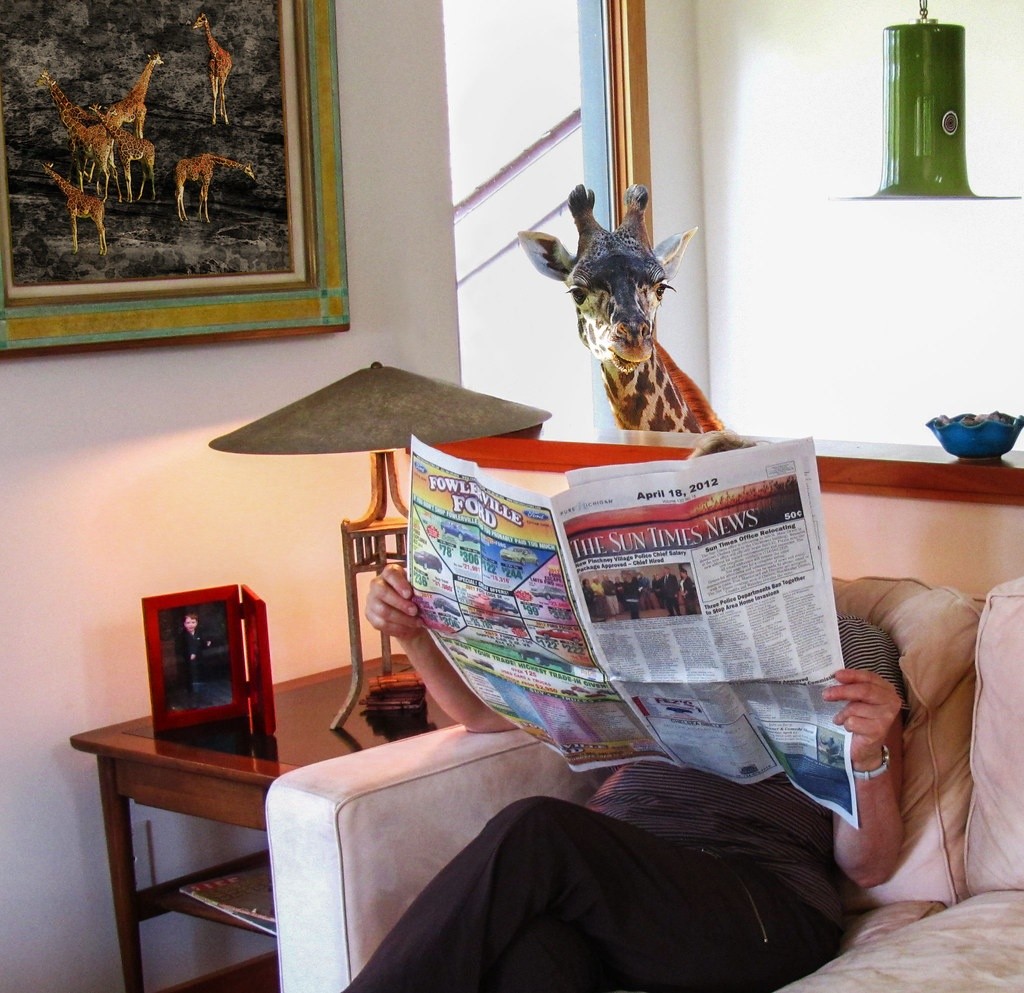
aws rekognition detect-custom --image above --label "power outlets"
[129,819,154,892]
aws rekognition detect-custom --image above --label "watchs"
[854,745,892,781]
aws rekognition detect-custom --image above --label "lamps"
[204,360,552,731]
[836,0,1021,201]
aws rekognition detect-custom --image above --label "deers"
[516,183,724,436]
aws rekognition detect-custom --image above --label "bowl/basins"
[925,410,1024,461]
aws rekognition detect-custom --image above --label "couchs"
[264,576,1024,993]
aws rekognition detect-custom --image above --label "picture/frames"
[141,585,278,737]
[0,0,350,361]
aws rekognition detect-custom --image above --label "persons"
[582,568,698,622]
[340,433,909,993]
[177,613,214,708]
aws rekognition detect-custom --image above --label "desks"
[405,426,1024,508]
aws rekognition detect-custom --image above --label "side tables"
[72,653,466,992]
